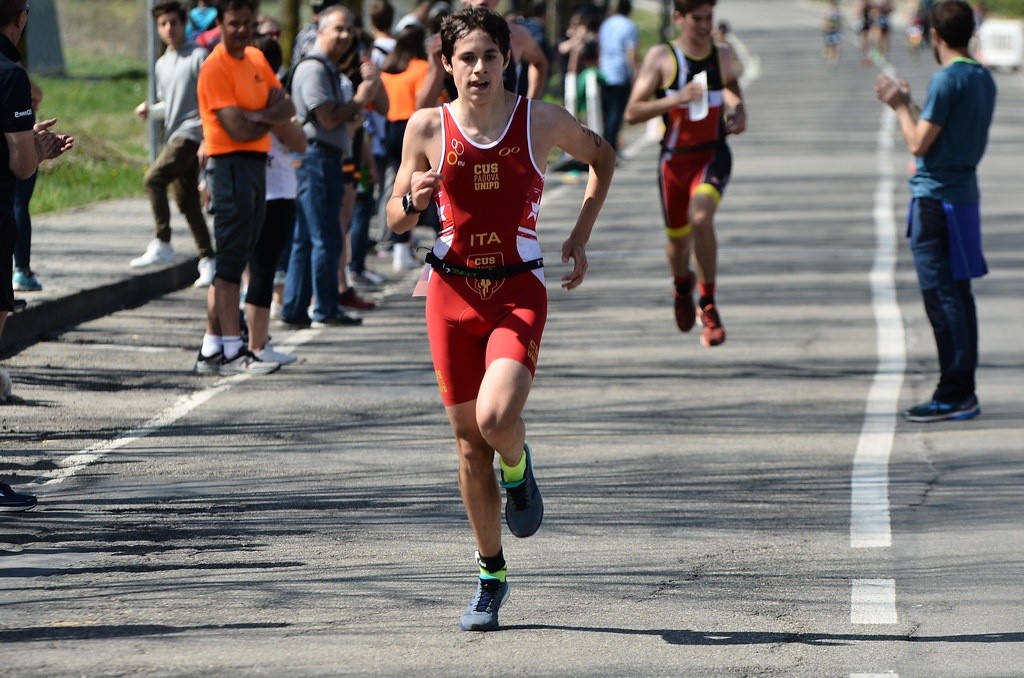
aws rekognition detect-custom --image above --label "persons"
[134,0,638,376]
[823,0,984,68]
[876,0,997,423]
[0,0,76,512]
[623,1,746,347]
[385,4,617,629]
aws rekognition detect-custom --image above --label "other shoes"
[378,249,393,259]
[240,327,272,342]
[255,342,298,367]
[349,268,387,286]
[277,316,312,330]
[671,270,697,331]
[308,311,363,329]
[270,301,285,319]
[7,299,27,312]
[337,287,376,312]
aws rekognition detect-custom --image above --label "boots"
[392,243,423,272]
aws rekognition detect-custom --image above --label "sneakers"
[194,345,224,375]
[218,344,281,375]
[904,392,982,422]
[0,483,37,512]
[695,303,726,347]
[12,266,43,292]
[499,441,544,539]
[194,257,217,287]
[130,237,175,268]
[460,568,510,632]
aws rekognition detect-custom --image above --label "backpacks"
[279,55,341,128]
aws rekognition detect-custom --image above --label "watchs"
[402,191,422,216]
